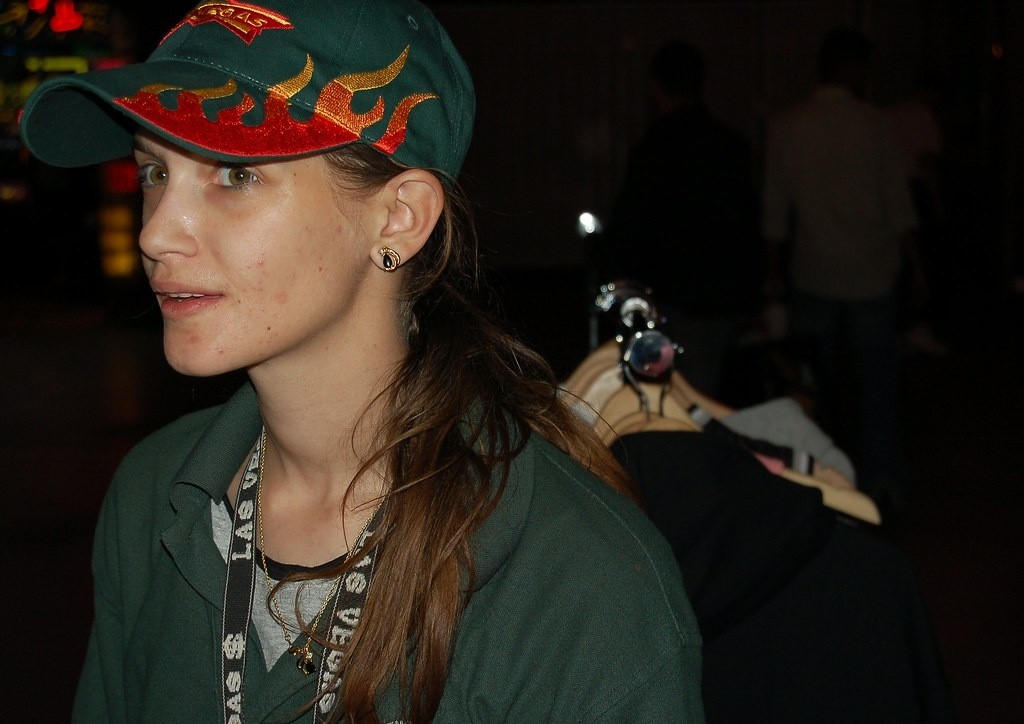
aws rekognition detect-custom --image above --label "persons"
[18,0,705,724]
[585,25,941,511]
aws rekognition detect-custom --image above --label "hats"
[20,0,477,194]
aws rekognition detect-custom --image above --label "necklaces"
[257,420,392,676]
